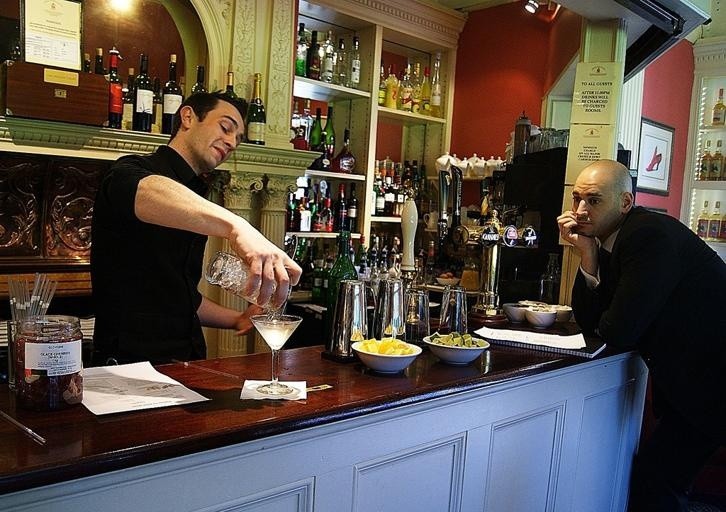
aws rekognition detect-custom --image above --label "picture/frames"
[635,116,675,196]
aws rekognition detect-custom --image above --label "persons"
[85,86,304,365]
[556,159,724,510]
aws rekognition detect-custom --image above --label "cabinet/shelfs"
[284,0,470,304]
[679,35,725,264]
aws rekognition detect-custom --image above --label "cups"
[532,129,569,149]
[204,252,292,319]
[403,289,430,349]
[437,285,468,335]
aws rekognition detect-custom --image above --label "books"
[481,326,608,361]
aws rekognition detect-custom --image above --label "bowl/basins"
[350,339,422,374]
[502,300,573,330]
[421,334,490,365]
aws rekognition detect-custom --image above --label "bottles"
[695,201,708,241]
[711,88,725,124]
[710,140,723,181]
[511,117,530,156]
[83,47,266,145]
[707,201,721,242]
[538,252,560,305]
[699,141,711,181]
[281,21,443,300]
[718,214,725,242]
[368,278,404,341]
[325,279,367,355]
[10,315,83,409]
[322,231,358,327]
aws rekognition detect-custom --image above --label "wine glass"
[250,314,302,395]
